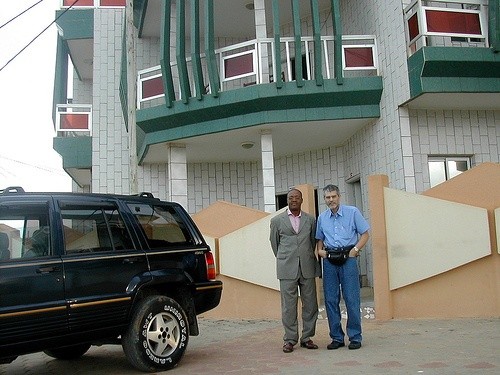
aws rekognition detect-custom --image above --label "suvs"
[0,186,223,371]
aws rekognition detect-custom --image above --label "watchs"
[354,247,360,252]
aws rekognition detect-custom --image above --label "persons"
[269,188,322,354]
[313,185,370,350]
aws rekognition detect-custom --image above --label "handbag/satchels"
[326,245,355,266]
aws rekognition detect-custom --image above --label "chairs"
[21,228,49,258]
[0,232,11,261]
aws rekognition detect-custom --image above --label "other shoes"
[300,340,318,349]
[327,341,345,349]
[282,342,293,353]
[348,341,362,350]
